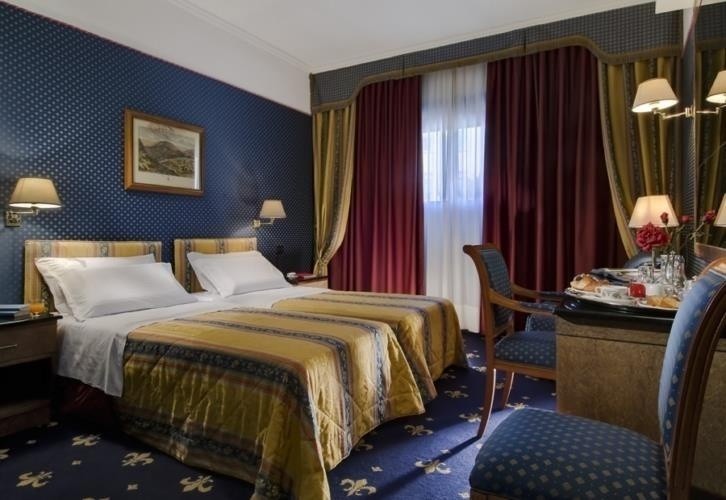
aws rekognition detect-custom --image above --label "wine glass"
[28,297,45,317]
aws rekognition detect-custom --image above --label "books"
[0,303,30,311]
[294,272,316,281]
[0,312,32,320]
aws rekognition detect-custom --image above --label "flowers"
[636,210,717,268]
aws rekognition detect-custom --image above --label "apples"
[631,284,646,297]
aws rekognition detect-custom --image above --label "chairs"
[462,242,567,435]
[469,255,725,498]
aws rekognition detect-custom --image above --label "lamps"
[693,70,726,114]
[631,78,693,121]
[4,176,59,227]
[712,193,726,229]
[627,193,679,284]
[252,198,285,228]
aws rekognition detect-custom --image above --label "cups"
[637,265,654,283]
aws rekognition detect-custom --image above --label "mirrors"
[691,0,726,260]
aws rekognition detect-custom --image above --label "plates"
[566,284,681,311]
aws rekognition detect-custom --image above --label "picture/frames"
[124,110,203,196]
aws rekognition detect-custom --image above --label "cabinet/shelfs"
[556,294,725,497]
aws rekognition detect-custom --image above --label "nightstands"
[285,274,329,289]
[0,309,62,440]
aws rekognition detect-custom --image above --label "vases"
[659,254,688,299]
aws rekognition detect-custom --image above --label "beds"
[175,237,470,402]
[24,240,425,498]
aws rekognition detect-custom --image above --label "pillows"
[34,252,156,321]
[186,251,265,292]
[64,263,197,322]
[196,255,289,298]
